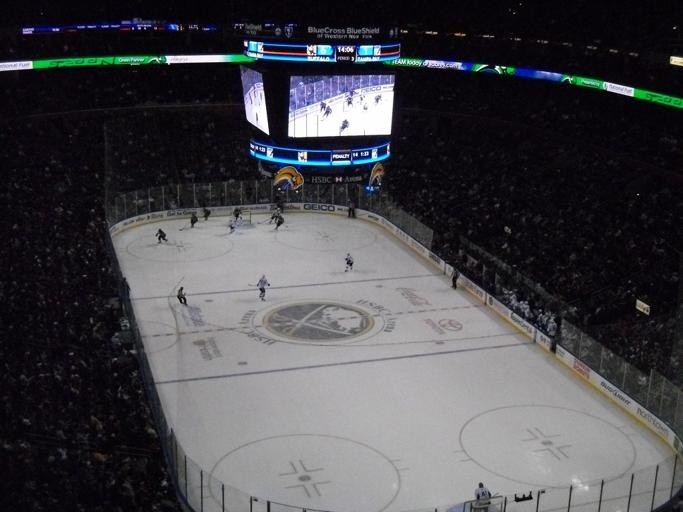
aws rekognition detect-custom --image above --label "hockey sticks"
[179,222,191,231]
[257,218,271,224]
[248,283,258,286]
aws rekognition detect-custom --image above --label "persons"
[344,252,354,273]
[0,0,381,214]
[256,274,269,302]
[189,214,197,228]
[269,207,279,224]
[381,0,683,443]
[2,215,186,511]
[202,207,210,221]
[346,199,355,219]
[273,214,284,232]
[475,482,491,510]
[231,207,241,221]
